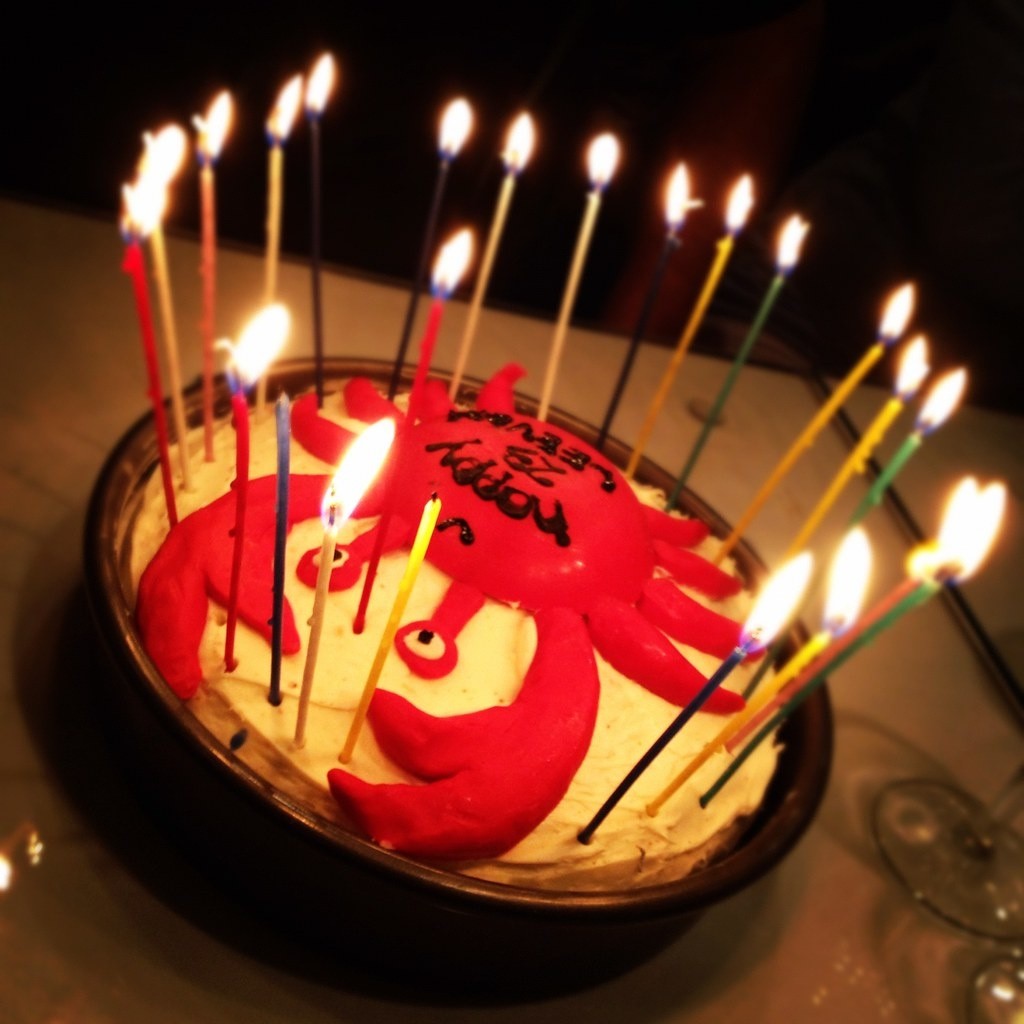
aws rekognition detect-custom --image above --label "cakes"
[137,385,779,889]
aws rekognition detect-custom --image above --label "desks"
[0,196,1023,1024]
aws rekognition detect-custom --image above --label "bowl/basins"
[88,358,833,973]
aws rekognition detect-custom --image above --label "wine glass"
[872,762,1024,942]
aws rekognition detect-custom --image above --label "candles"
[594,161,703,451]
[647,477,1008,819]
[784,335,929,555]
[447,112,536,403]
[387,98,475,401]
[576,550,814,846]
[838,367,968,528]
[712,281,917,568]
[269,393,291,706]
[304,52,338,408]
[539,133,624,423]
[354,227,474,633]
[622,172,755,479]
[192,91,237,464]
[663,214,812,513]
[337,493,442,763]
[118,125,198,527]
[295,416,398,742]
[254,74,303,423]
[209,302,291,673]
